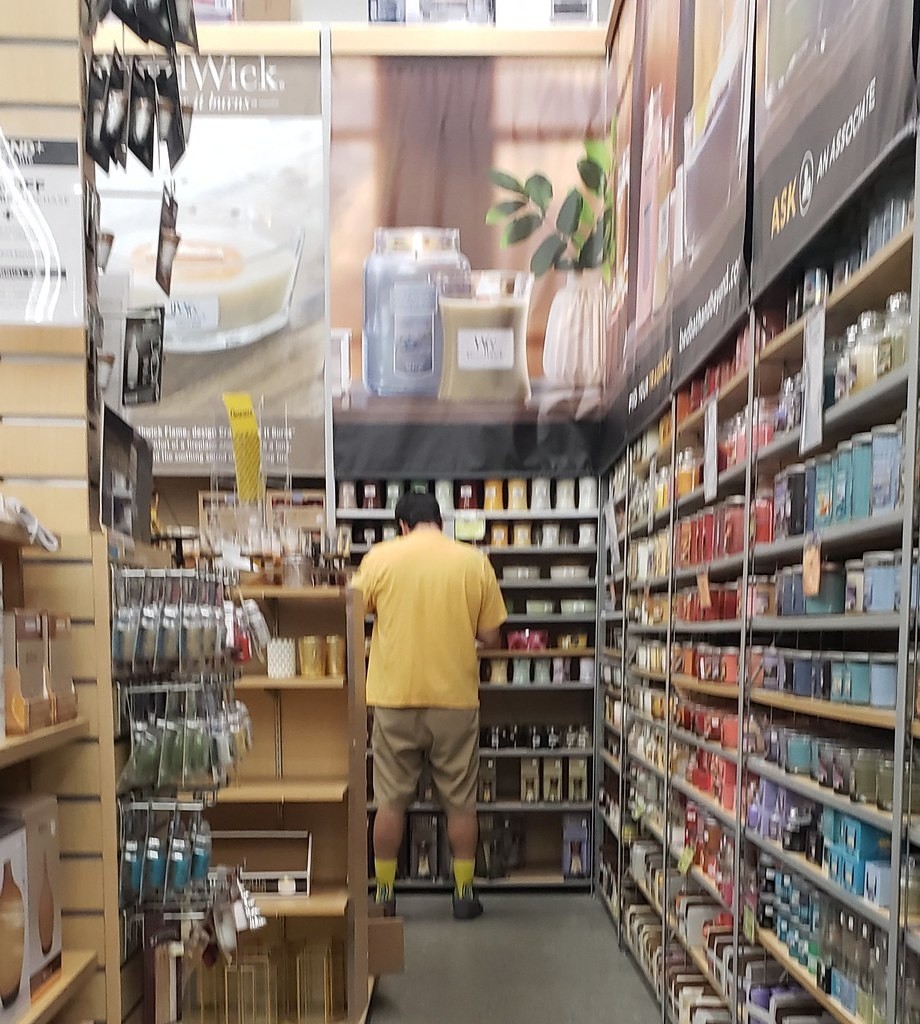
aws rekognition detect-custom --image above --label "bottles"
[37,849,57,956]
[362,224,471,398]
[127,325,137,390]
[336,476,597,879]
[0,859,25,1008]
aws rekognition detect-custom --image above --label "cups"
[611,58,703,336]
[430,266,536,402]
[369,0,407,22]
[326,635,347,675]
[597,192,919,1024]
[282,553,321,590]
[266,637,296,678]
[297,635,327,676]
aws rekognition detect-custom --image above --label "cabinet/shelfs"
[0,137,920,1023]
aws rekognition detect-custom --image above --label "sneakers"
[455,891,484,919]
[376,898,397,919]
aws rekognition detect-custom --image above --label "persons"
[344,491,509,921]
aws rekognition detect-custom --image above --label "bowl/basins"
[109,198,306,354]
